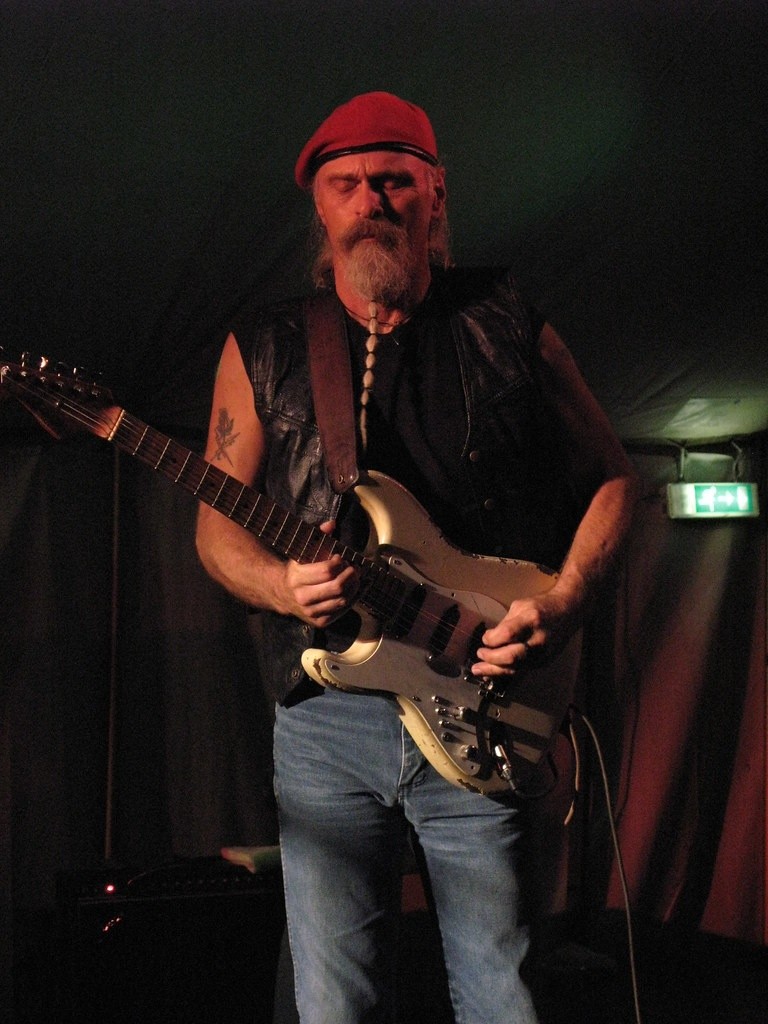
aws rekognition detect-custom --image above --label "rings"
[523,642,532,654]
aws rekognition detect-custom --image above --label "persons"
[195,89,643,1024]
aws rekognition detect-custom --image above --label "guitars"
[0,349,590,799]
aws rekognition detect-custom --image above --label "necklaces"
[334,288,435,346]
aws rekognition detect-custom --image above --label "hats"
[295,91,439,191]
